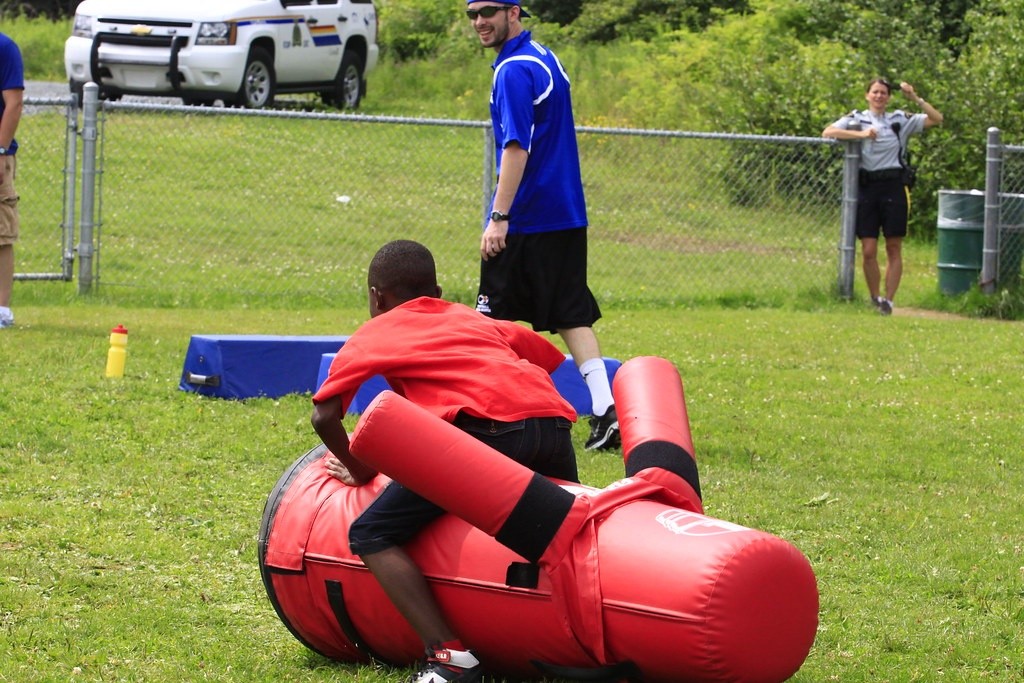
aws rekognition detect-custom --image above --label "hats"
[468,0,531,19]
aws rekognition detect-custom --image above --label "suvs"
[65,0,376,108]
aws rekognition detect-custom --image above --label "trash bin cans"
[937,188,1024,298]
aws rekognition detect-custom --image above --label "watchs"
[0,146,8,156]
[487,210,510,221]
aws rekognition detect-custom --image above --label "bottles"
[105,324,128,377]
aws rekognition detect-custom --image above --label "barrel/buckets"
[937,190,1024,298]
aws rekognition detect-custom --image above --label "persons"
[466,0,622,450]
[0,32,25,329]
[312,238,580,683]
[821,77,944,316]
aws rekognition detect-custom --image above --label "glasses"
[466,6,508,19]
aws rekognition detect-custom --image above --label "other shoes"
[869,300,895,317]
[0,308,15,328]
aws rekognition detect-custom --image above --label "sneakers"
[406,652,495,682]
[585,404,622,451]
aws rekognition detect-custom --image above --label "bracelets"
[916,98,924,105]
